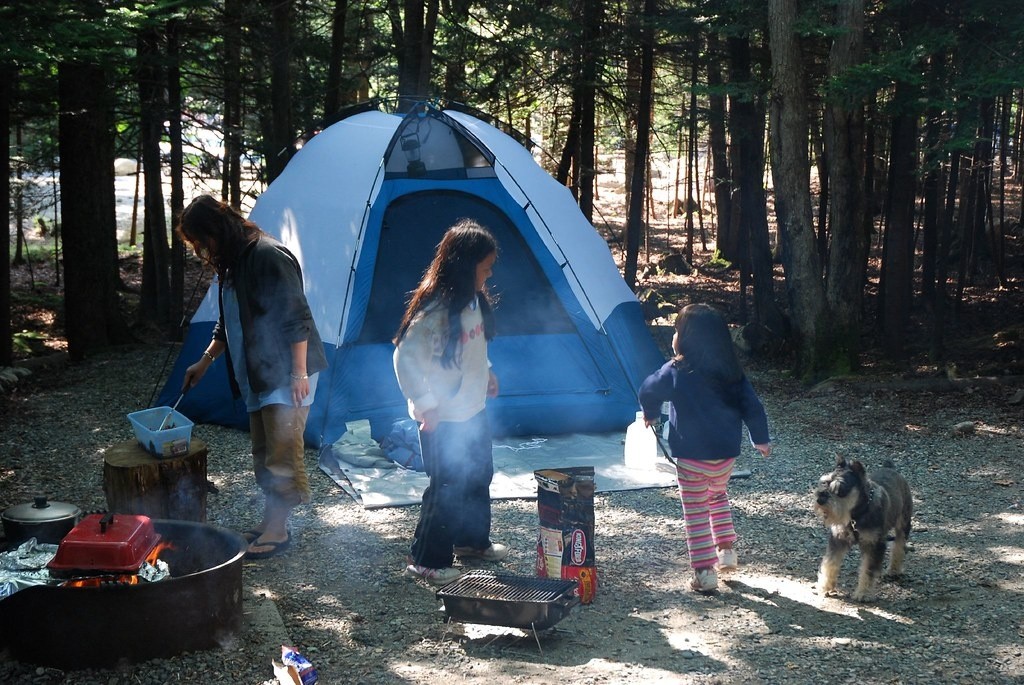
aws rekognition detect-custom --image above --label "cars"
[159,127,264,179]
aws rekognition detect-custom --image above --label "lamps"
[401,133,428,178]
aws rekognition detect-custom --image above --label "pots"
[1,493,81,551]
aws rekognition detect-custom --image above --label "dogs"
[811,451,914,603]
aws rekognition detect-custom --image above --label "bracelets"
[289,372,308,380]
[202,351,216,362]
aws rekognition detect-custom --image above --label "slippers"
[244,533,292,560]
[241,529,291,544]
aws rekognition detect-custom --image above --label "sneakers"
[715,546,737,571]
[453,543,508,562]
[406,560,461,585]
[689,569,719,592]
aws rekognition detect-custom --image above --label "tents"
[154,93,667,450]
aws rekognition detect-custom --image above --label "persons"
[392,223,509,585]
[180,195,330,560]
[638,303,772,594]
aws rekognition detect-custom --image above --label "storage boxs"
[127,405,194,459]
[532,464,597,604]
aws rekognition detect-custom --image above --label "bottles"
[625,410,657,470]
